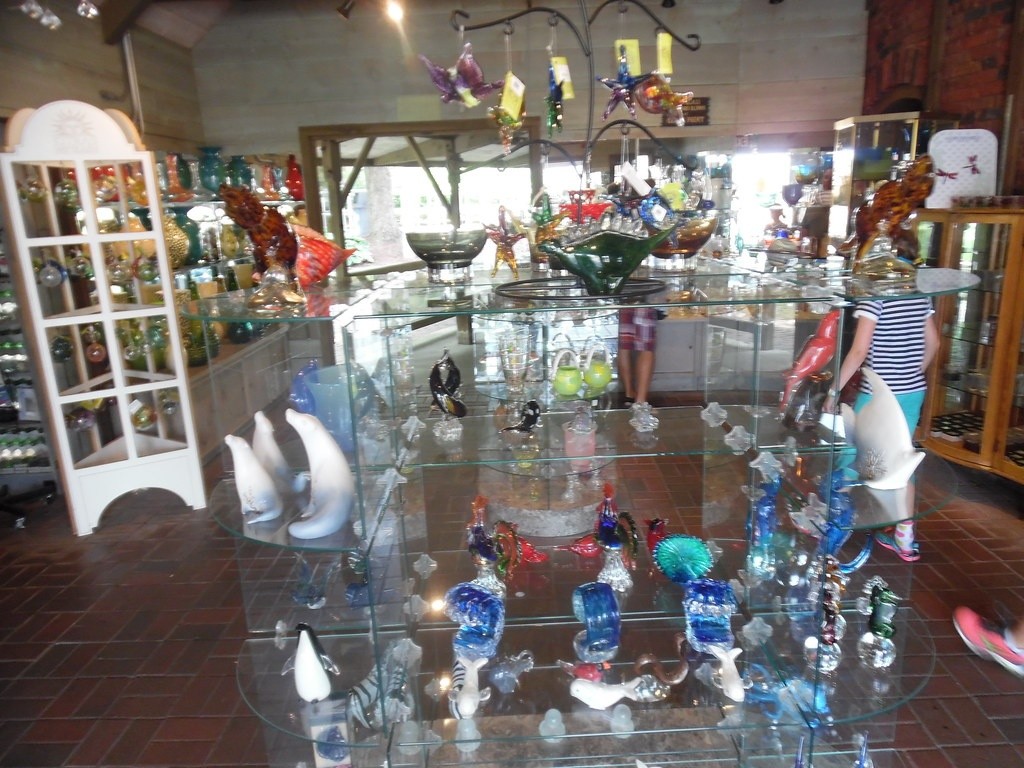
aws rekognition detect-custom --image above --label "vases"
[128,144,312,370]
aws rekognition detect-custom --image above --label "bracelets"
[827,387,841,399]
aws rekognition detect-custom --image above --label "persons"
[952,604,1024,675]
[617,305,657,409]
[820,229,941,563]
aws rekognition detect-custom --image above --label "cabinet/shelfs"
[0,97,206,536]
[181,261,981,767]
[911,209,1024,484]
[102,198,312,457]
[828,111,962,262]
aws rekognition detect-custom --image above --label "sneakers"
[788,510,828,543]
[875,526,921,561]
[953,604,1024,679]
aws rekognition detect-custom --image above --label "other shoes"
[623,396,636,409]
